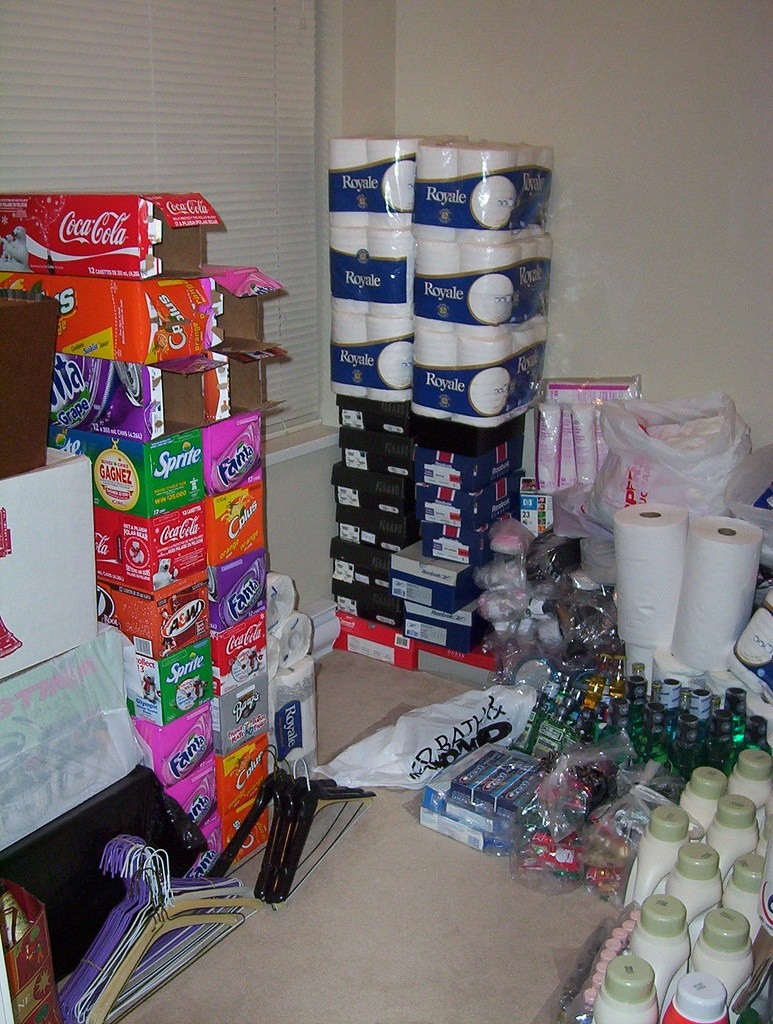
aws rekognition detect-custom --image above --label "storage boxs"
[214,733,270,814]
[0,445,97,681]
[0,762,207,983]
[203,346,289,420]
[220,795,269,867]
[197,815,221,855]
[47,351,229,443]
[208,547,267,633]
[95,570,211,661]
[0,294,60,479]
[0,627,142,854]
[204,480,264,566]
[0,876,62,1024]
[131,700,215,789]
[328,395,554,694]
[210,670,269,756]
[46,426,204,520]
[126,635,214,726]
[210,610,267,698]
[197,263,285,357]
[201,411,263,495]
[0,271,206,365]
[164,751,217,829]
[0,191,224,281]
[91,497,207,594]
[419,742,545,855]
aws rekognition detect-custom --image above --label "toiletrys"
[591,748,771,1023]
[415,741,549,855]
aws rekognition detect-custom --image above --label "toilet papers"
[329,137,554,428]
[671,515,762,674]
[615,502,689,649]
[624,588,772,750]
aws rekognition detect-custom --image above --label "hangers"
[56,745,379,1024]
